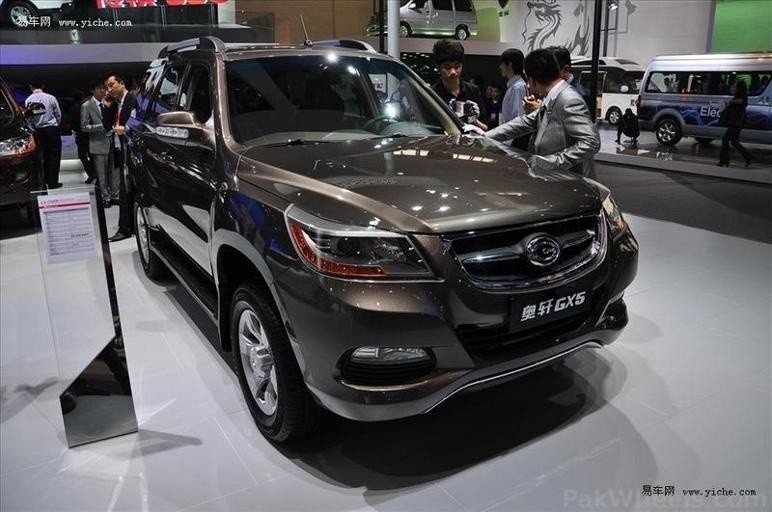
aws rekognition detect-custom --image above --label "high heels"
[745,153,756,167]
[716,159,730,168]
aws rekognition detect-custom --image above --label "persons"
[544,44,593,114]
[70,90,94,183]
[488,85,501,108]
[80,81,121,208]
[664,78,671,92]
[616,107,640,145]
[461,49,599,181]
[100,76,138,242]
[498,48,530,145]
[430,40,489,132]
[23,74,62,189]
[716,79,755,169]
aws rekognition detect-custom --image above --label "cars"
[0,0,74,27]
[0,76,49,231]
[122,14,640,446]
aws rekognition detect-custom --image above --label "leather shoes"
[104,196,120,208]
[84,174,96,184]
[108,228,132,241]
[48,182,63,189]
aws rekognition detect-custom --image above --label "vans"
[366,0,479,41]
[566,51,772,147]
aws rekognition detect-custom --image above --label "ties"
[533,105,547,129]
[99,103,105,117]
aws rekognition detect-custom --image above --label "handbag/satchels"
[718,109,745,128]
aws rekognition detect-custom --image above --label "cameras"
[460,102,473,115]
